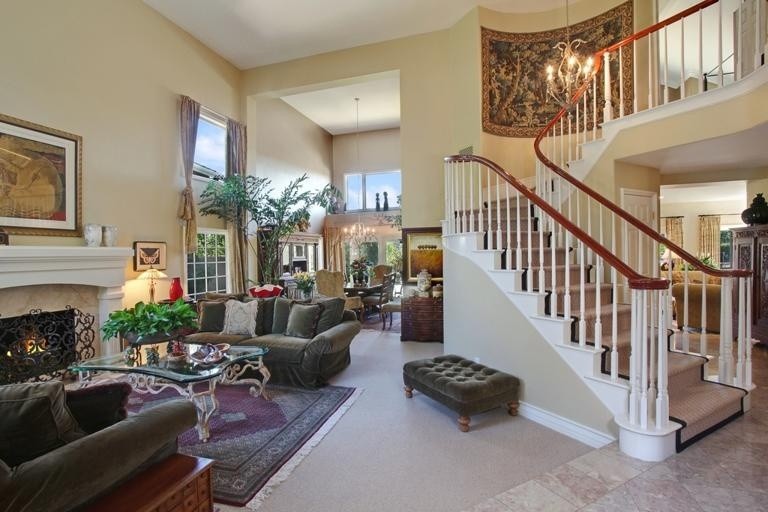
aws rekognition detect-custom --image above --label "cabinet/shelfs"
[728,224,768,342]
[0,245,136,391]
[400,297,443,342]
[255,229,322,287]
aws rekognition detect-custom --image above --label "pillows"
[195,292,347,340]
[0,381,89,467]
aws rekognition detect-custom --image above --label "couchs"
[672,282,721,335]
[178,309,362,391]
[401,354,521,434]
[660,270,721,321]
[0,379,218,512]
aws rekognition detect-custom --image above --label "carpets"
[360,311,401,334]
[76,374,367,512]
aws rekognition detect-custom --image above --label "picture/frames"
[400,226,443,286]
[132,240,169,273]
[0,111,85,239]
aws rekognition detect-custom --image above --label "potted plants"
[95,297,200,366]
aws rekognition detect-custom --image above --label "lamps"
[134,265,168,305]
[542,1,596,121]
[341,97,377,238]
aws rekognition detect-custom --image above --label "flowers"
[345,256,376,279]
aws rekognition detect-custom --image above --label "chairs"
[248,284,283,298]
[312,263,401,331]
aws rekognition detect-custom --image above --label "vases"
[169,277,184,301]
[353,273,369,286]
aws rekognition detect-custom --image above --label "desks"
[157,297,194,305]
